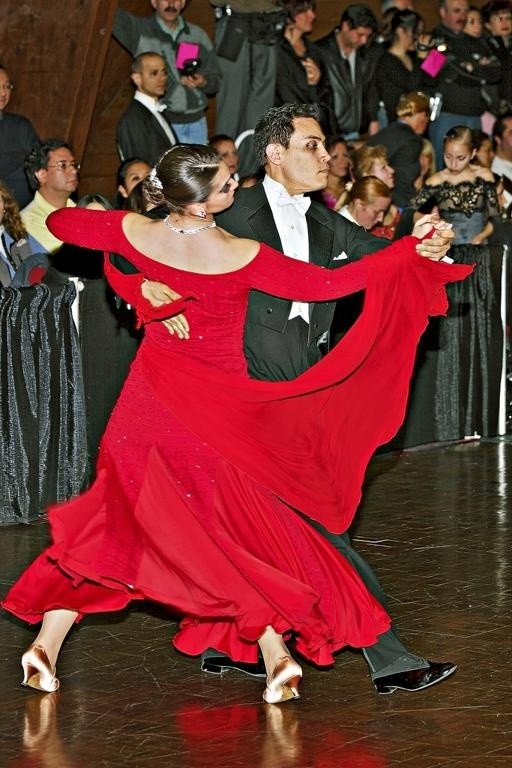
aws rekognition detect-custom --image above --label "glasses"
[45,162,81,171]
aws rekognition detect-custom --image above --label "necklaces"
[163,212,218,235]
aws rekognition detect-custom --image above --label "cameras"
[176,57,201,83]
[430,36,448,53]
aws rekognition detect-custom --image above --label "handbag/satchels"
[217,16,248,62]
[248,11,286,46]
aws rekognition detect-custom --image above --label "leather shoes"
[374,660,456,694]
[201,655,266,677]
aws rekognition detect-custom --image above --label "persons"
[115,1,512,457]
[107,100,457,695]
[0,184,94,528]
[55,194,146,495]
[1,63,42,182]
[124,174,159,214]
[19,137,78,256]
[1,143,479,704]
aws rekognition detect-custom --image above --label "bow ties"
[278,191,311,215]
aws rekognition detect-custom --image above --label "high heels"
[22,644,59,692]
[262,656,302,704]
[22,693,57,753]
[262,705,300,760]
